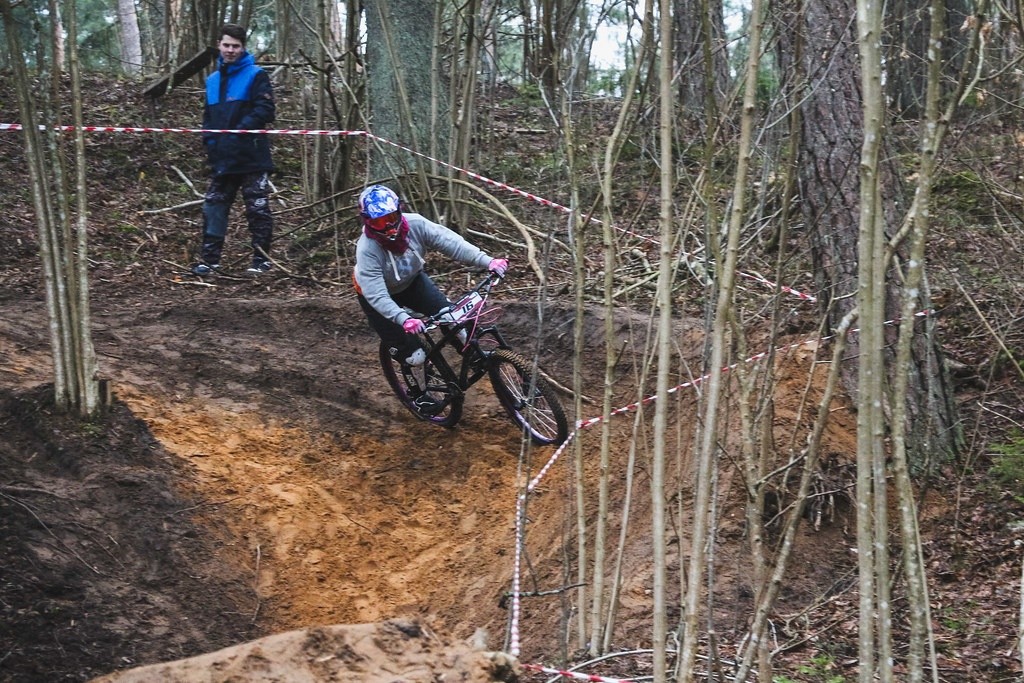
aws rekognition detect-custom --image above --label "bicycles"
[376,251,571,450]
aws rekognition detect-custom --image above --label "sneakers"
[248,257,273,275]
[471,350,495,358]
[193,263,222,276]
[412,393,444,414]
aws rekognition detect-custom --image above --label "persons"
[193,24,276,275]
[352,184,508,407]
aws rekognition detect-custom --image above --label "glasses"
[368,210,401,229]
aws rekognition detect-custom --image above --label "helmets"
[358,184,407,238]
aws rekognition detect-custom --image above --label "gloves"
[403,319,426,336]
[489,257,508,278]
